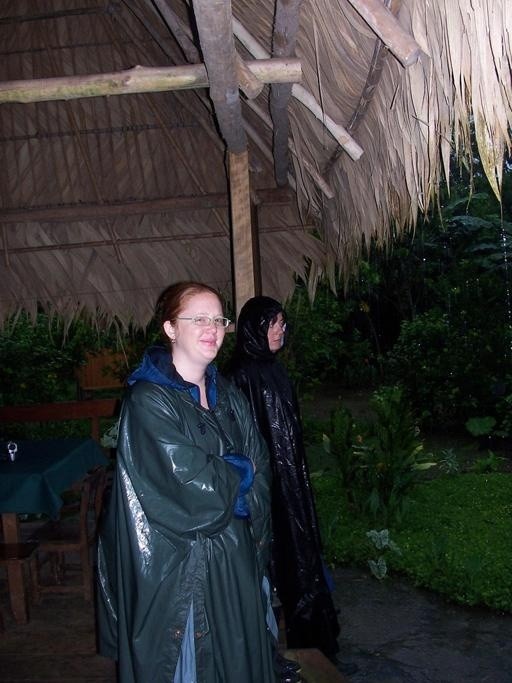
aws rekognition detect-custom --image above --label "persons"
[94,281,277,683]
[270,644,304,683]
[219,295,358,675]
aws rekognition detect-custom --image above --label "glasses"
[171,315,232,329]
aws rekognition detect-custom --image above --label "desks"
[0,437,109,626]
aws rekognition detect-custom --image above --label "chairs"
[0,542,43,625]
[28,467,107,600]
[41,463,115,603]
